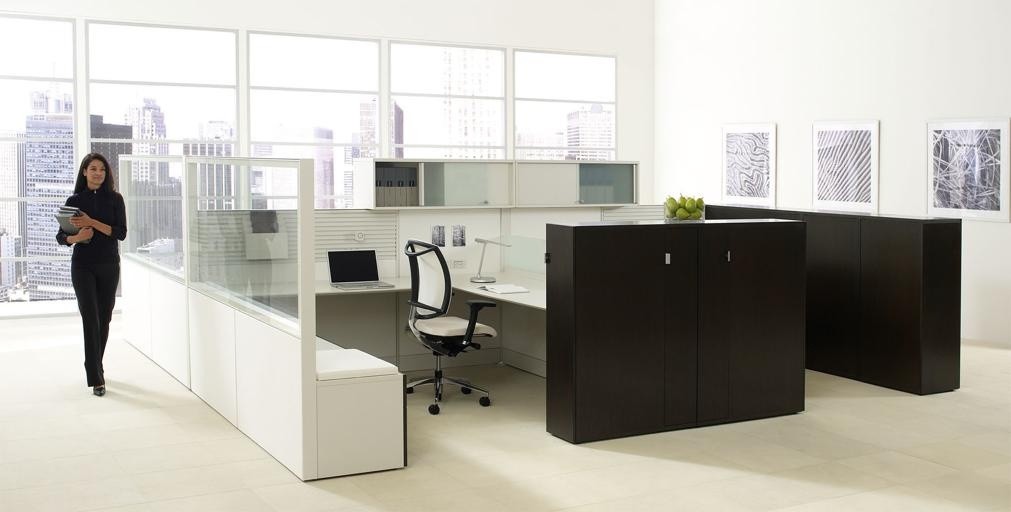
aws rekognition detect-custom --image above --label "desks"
[222,274,547,380]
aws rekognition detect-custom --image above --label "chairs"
[402,238,499,414]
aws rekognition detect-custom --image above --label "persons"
[56,152,127,396]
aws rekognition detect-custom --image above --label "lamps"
[470,237,512,283]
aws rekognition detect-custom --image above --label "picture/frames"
[720,115,1010,223]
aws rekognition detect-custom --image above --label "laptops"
[327,249,394,290]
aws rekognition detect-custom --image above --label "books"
[479,285,528,294]
[56,206,92,244]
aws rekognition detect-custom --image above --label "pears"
[665,192,704,219]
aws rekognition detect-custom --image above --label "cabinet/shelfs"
[705,203,962,395]
[543,219,808,444]
[353,158,639,211]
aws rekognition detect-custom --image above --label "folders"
[375,167,419,207]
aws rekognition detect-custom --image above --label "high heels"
[93,383,106,396]
